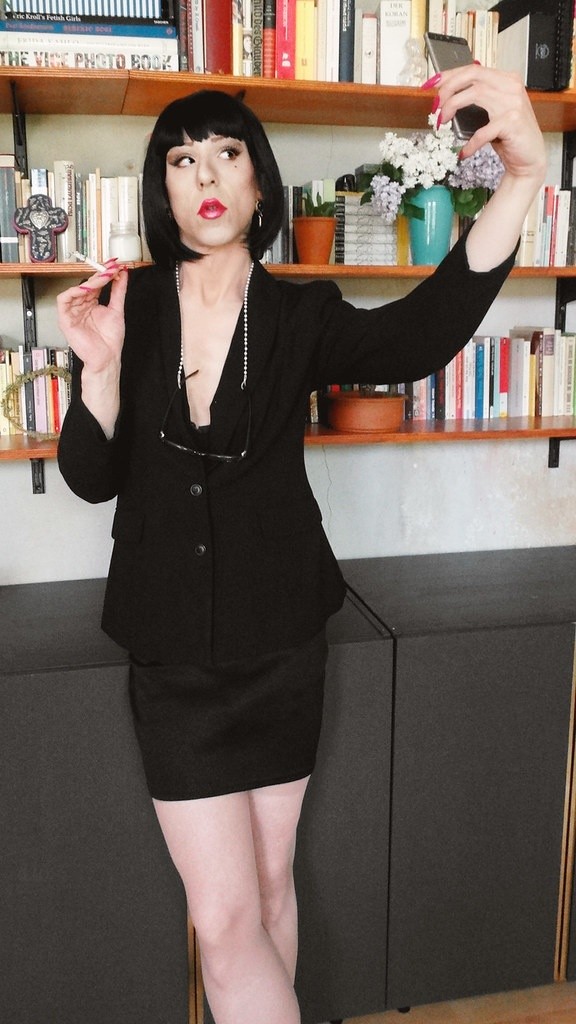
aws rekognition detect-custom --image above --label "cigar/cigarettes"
[72,249,122,282]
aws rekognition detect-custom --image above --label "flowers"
[369,103,507,224]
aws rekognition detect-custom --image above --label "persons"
[55,36,552,1024]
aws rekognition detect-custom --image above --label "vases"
[403,183,457,268]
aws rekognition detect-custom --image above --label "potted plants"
[289,192,340,264]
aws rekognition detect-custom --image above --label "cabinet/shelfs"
[1,65,576,477]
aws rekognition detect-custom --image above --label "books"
[0,1,574,437]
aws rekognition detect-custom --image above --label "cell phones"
[424,31,500,141]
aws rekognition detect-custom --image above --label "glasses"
[158,369,252,462]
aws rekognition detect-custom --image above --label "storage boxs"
[2,554,393,1024]
[338,546,575,1012]
